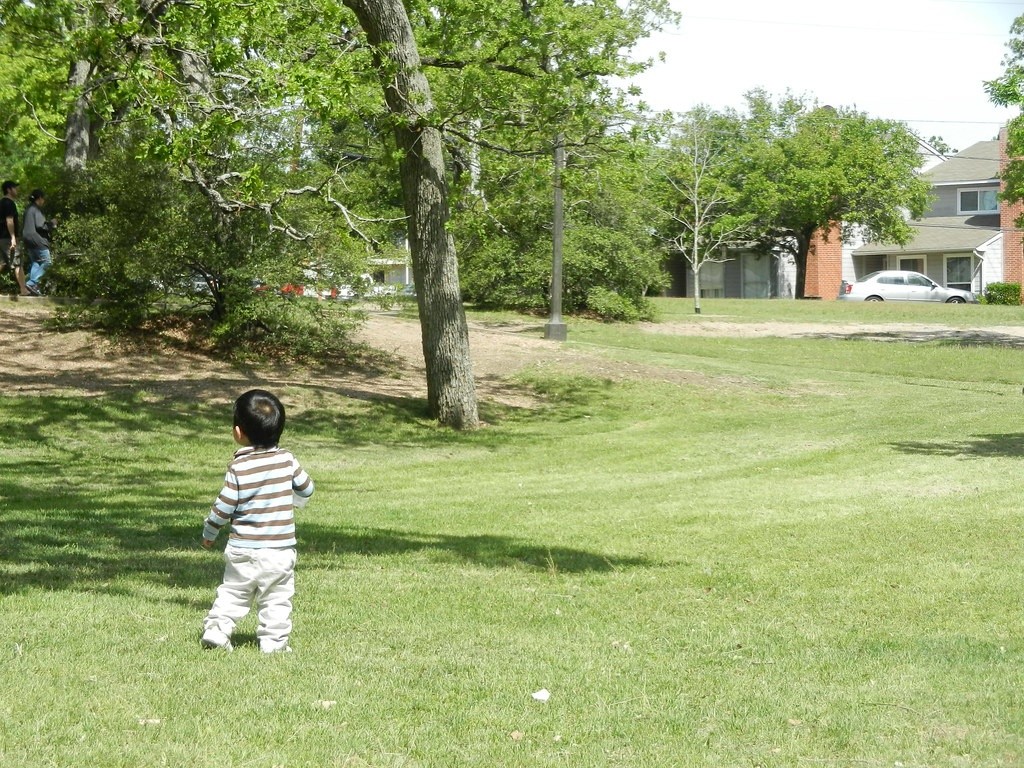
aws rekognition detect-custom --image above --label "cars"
[840,270,977,303]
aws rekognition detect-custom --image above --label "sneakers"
[199,632,233,654]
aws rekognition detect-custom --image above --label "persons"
[201,389,314,654]
[22,189,58,297]
[1,181,35,296]
